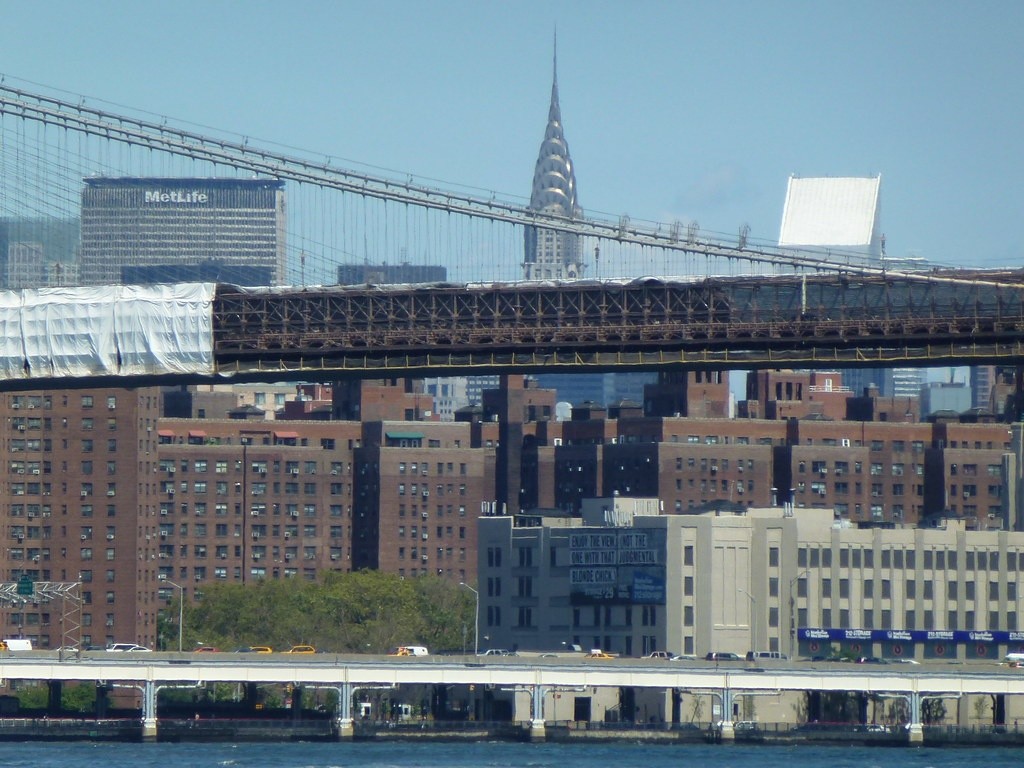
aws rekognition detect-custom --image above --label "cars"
[0,639,1024,668]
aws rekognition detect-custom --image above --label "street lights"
[161,579,183,653]
[738,588,758,651]
[459,582,479,656]
